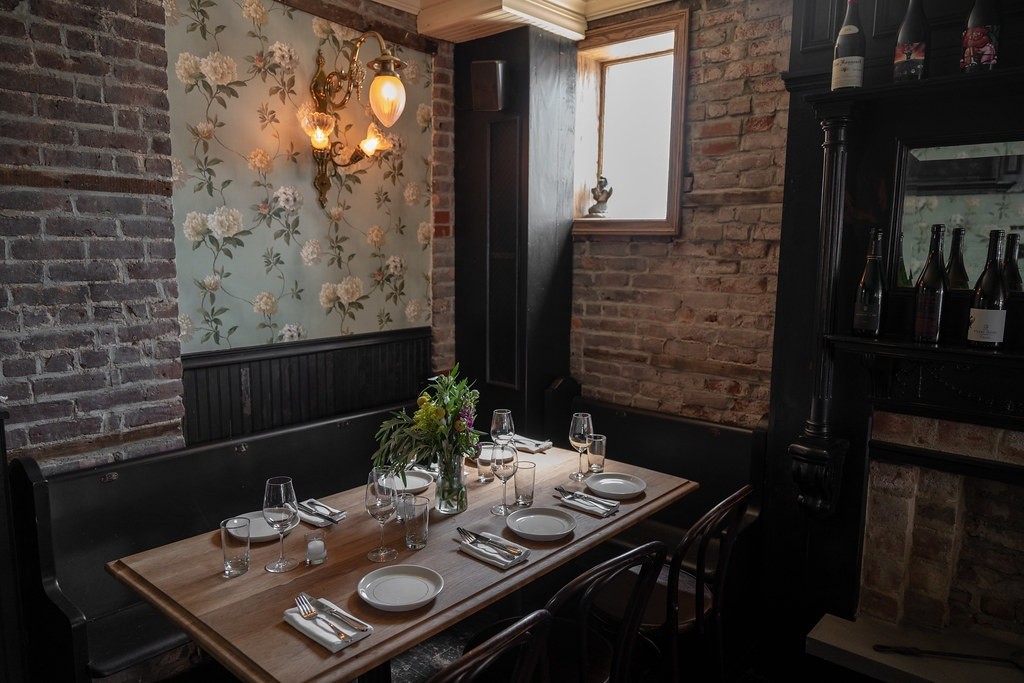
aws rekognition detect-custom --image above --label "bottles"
[851,223,1010,353]
[899,232,1021,291]
[832,0,1008,93]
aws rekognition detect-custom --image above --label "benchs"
[545,377,770,596]
[10,395,433,683]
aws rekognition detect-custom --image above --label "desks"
[105,436,698,683]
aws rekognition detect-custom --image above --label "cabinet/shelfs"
[785,55,1024,514]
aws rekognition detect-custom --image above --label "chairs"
[391,483,755,683]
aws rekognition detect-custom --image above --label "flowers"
[370,362,488,510]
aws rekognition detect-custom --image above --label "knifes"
[554,487,617,506]
[466,531,524,555]
[298,592,369,632]
[298,504,338,525]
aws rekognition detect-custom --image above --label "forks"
[457,526,517,560]
[557,486,611,512]
[307,501,345,517]
[295,596,354,643]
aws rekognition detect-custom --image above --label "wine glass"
[365,469,399,562]
[569,413,593,482]
[262,476,299,572]
[490,408,519,517]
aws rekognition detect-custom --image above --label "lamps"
[303,30,407,207]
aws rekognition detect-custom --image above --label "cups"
[374,465,430,549]
[477,442,536,507]
[585,434,606,473]
[220,517,328,578]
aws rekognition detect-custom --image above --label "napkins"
[284,597,373,653]
[508,431,552,453]
[283,497,347,527]
[560,490,621,518]
[459,531,532,570]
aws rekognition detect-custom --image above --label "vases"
[436,451,467,514]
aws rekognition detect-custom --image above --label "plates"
[470,444,515,462]
[586,472,647,499]
[377,470,433,494]
[228,510,300,542]
[506,508,577,541]
[357,564,443,612]
